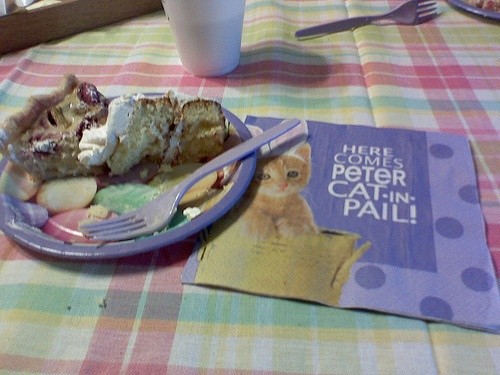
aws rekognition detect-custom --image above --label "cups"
[163,0,246,77]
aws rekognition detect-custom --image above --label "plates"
[446,0,500,18]
[0,93,257,260]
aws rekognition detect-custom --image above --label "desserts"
[0,74,227,176]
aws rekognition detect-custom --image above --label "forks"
[294,0,438,39]
[74,120,301,241]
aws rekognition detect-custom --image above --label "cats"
[227,141,321,238]
